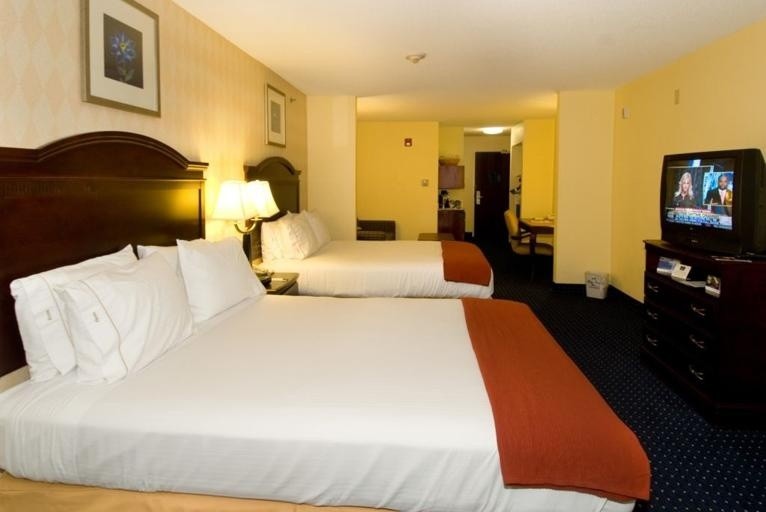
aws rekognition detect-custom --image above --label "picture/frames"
[79,1,160,117]
[265,82,286,148]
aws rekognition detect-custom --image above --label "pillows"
[260,209,330,263]
[9,235,268,386]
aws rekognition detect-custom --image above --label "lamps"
[211,180,280,269]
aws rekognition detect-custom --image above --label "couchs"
[357,217,395,241]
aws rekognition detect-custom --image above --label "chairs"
[503,207,553,257]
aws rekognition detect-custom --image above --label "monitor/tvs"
[659,148,766,258]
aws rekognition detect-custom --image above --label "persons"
[439,190,450,209]
[705,175,729,205]
[510,177,521,217]
[673,172,696,209]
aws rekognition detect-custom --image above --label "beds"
[0,130,651,512]
[243,156,494,299]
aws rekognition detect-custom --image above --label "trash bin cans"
[585,271,609,299]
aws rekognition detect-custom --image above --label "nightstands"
[254,272,299,296]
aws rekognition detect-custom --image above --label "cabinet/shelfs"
[640,240,766,428]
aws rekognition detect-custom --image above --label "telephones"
[252,264,269,276]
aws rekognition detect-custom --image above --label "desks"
[519,218,555,257]
[418,233,455,241]
